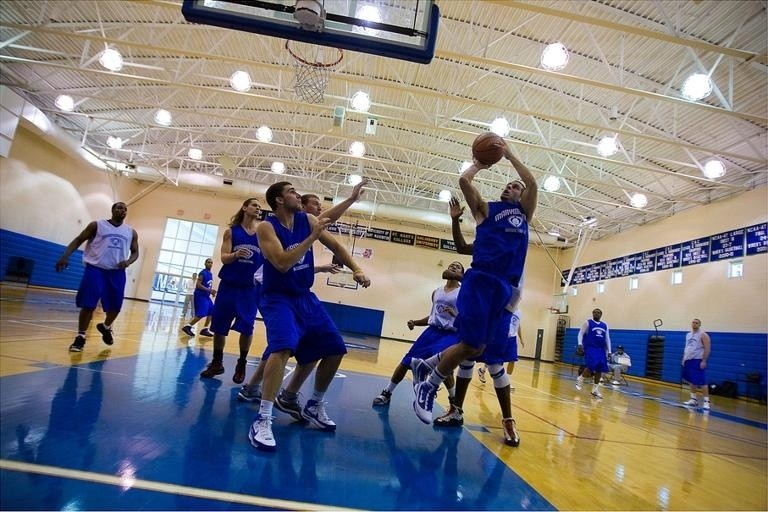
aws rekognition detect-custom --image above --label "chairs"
[606,367,630,386]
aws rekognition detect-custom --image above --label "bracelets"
[352,269,363,275]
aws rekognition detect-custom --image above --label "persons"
[200,197,266,383]
[432,198,521,448]
[248,182,371,451]
[373,262,464,407]
[237,179,368,421]
[576,305,632,400]
[57,202,139,351]
[409,136,539,425]
[182,258,216,337]
[682,319,711,411]
[181,273,197,319]
[478,305,526,392]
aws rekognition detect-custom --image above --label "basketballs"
[472,131,504,163]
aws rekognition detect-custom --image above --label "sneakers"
[200,328,213,337]
[575,377,582,391]
[683,399,697,406]
[68,335,85,352]
[590,390,601,397]
[702,401,709,410]
[96,323,112,345]
[372,357,519,446]
[182,326,194,336]
[200,363,335,451]
